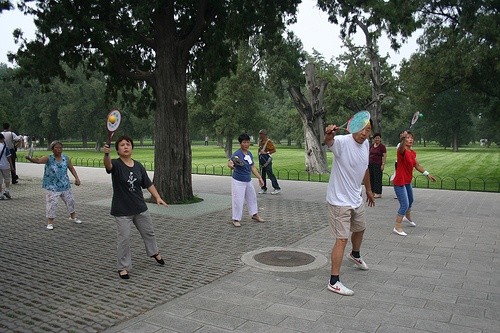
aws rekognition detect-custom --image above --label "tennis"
[109,116,116,124]
[419,114,423,119]
[365,120,370,127]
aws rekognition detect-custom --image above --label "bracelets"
[30,156,31,160]
[422,170,429,177]
[266,151,269,153]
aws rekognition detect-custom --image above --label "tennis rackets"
[407,111,420,133]
[230,155,245,171]
[104,109,122,158]
[327,109,371,135]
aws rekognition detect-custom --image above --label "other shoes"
[232,220,241,226]
[0,190,12,200]
[253,213,264,222]
[258,189,266,194]
[393,227,408,236]
[271,188,282,194]
[12,181,19,184]
[117,270,130,280]
[151,252,165,265]
[373,194,381,198]
[403,215,416,227]
[47,224,54,230]
[68,216,82,223]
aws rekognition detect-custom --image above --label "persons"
[97,137,102,152]
[205,133,208,145]
[0,123,22,183]
[324,117,375,296]
[369,133,386,197]
[104,136,168,279]
[258,129,282,194]
[0,133,12,200]
[394,130,437,236]
[227,134,264,226]
[24,135,37,157]
[25,139,82,229]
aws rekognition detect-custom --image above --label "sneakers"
[327,280,354,295]
[347,251,368,270]
